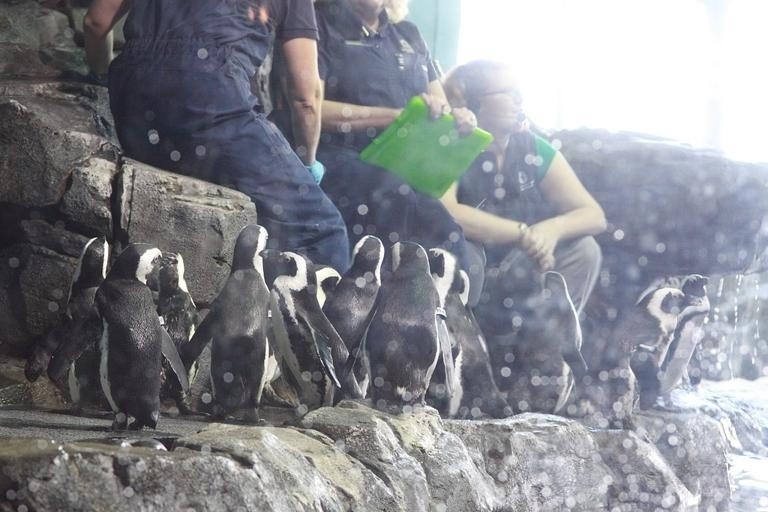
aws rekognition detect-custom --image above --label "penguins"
[23,222,517,435]
[596,286,702,432]
[656,274,712,414]
[506,269,590,414]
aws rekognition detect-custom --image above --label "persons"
[440,57,611,314]
[80,0,352,275]
[265,0,487,308]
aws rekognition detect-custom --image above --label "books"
[357,96,497,201]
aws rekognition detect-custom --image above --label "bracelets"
[518,222,528,241]
